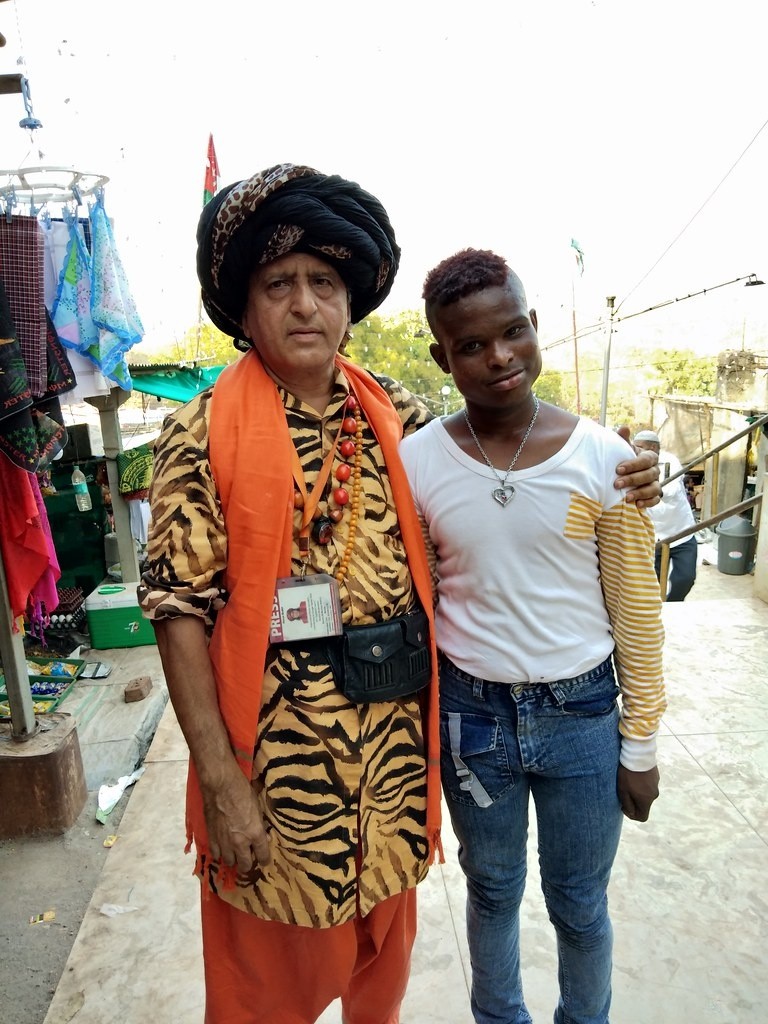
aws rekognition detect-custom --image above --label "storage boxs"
[84,582,158,650]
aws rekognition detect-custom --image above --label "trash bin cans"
[717,514,755,576]
[41,484,107,595]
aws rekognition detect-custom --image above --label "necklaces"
[336,397,362,584]
[464,395,538,508]
[294,395,356,545]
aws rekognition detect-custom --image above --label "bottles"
[71,466,92,512]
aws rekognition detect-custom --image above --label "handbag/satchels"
[324,606,432,702]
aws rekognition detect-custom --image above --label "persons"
[399,247,668,1024]
[135,165,663,1024]
[287,601,307,624]
[633,428,697,601]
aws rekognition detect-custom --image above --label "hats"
[195,164,402,344]
[633,430,660,443]
